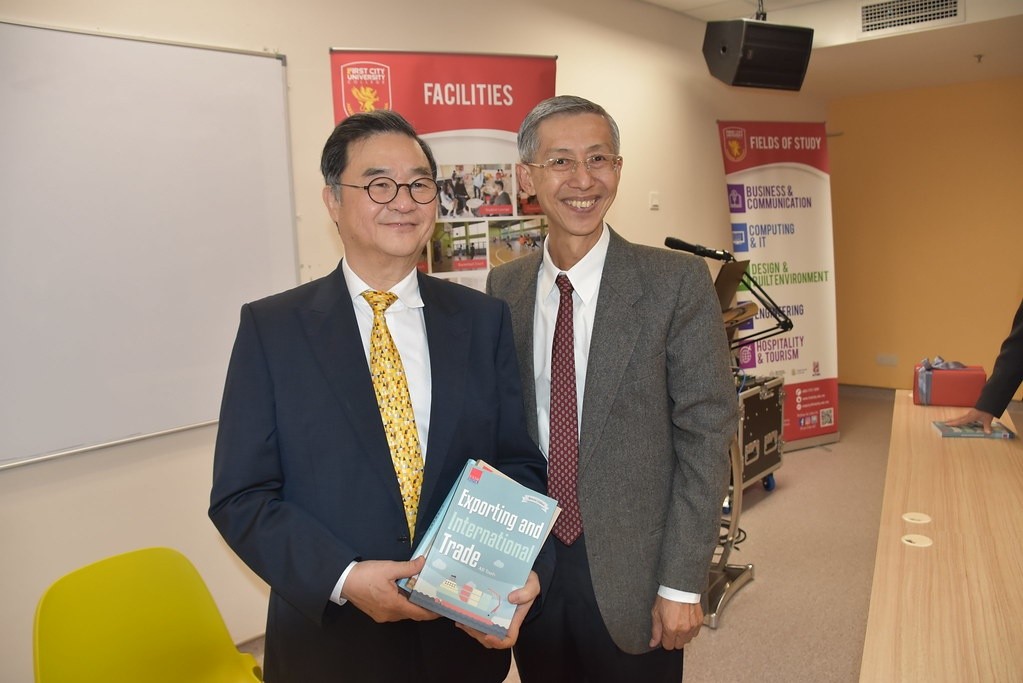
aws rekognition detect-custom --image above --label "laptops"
[713,260,751,313]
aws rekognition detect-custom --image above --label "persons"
[208,110,548,683]
[506,235,514,251]
[457,244,463,260]
[446,244,452,259]
[519,234,540,248]
[944,297,1023,435]
[473,166,483,200]
[470,243,475,260]
[496,169,505,180]
[490,180,513,216]
[490,95,741,683]
[440,170,471,218]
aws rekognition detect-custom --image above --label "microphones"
[663,236,731,263]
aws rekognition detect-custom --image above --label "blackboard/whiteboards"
[0,21,301,473]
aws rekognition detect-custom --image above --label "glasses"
[334,177,441,204]
[528,153,619,173]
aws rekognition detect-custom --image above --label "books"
[396,459,562,642]
[932,420,1016,439]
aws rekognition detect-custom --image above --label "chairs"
[32,546,263,683]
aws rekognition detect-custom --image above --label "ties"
[361,290,424,548]
[546,275,582,546]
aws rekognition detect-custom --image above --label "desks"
[859,389,1023,683]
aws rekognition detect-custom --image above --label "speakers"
[703,18,815,92]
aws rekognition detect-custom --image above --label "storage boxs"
[913,363,987,407]
[737,374,785,487]
